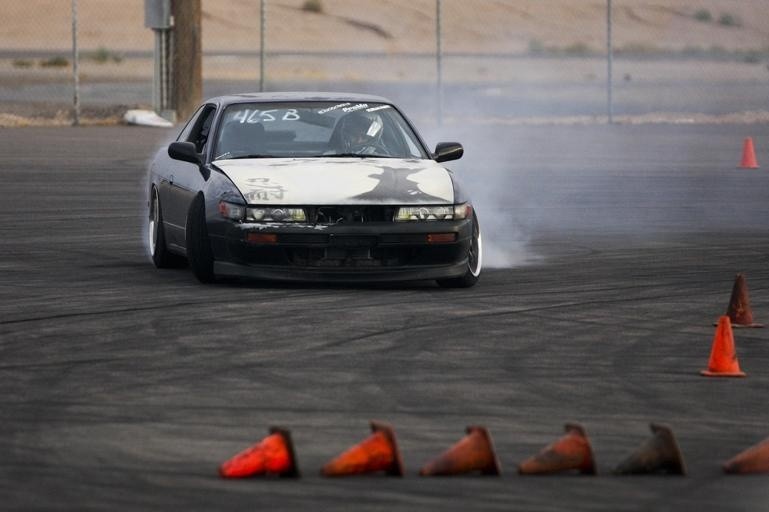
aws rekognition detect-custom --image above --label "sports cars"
[145,87,483,290]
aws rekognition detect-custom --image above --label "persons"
[318,110,401,159]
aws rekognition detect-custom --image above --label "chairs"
[222,119,266,157]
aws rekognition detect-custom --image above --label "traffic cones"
[217,422,299,481]
[609,420,687,476]
[721,434,768,476]
[517,423,601,479]
[317,420,403,478]
[422,425,503,479]
[736,136,762,170]
[700,315,747,379]
[709,269,765,329]
